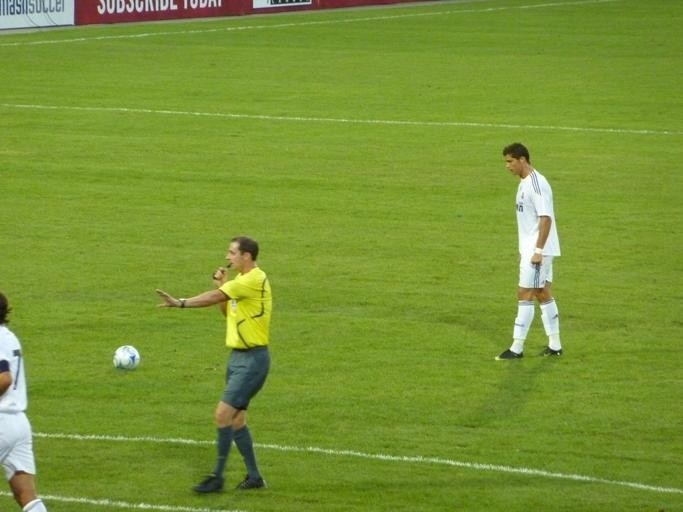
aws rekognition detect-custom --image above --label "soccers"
[112,346,140,371]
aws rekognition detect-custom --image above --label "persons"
[494,142,562,361]
[1,294,50,512]
[156,238,272,492]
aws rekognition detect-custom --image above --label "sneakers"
[494,350,523,360]
[539,348,562,357]
[192,472,224,492]
[237,475,264,488]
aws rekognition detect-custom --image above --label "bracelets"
[179,297,186,308]
[535,247,543,255]
